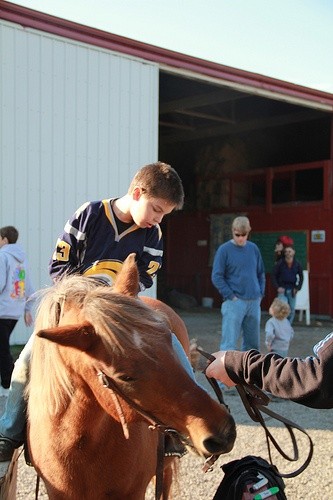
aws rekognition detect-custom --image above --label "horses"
[22,252,238,500]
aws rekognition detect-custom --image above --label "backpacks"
[211,454,287,500]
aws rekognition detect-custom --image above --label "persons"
[0,162,195,479]
[0,225,37,397]
[270,245,304,326]
[263,297,294,403]
[206,332,333,410]
[211,216,267,396]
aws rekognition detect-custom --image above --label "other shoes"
[0,437,16,463]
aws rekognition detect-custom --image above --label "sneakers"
[0,386,9,398]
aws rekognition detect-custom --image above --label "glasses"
[235,233,247,237]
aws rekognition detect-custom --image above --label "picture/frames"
[310,229,326,244]
